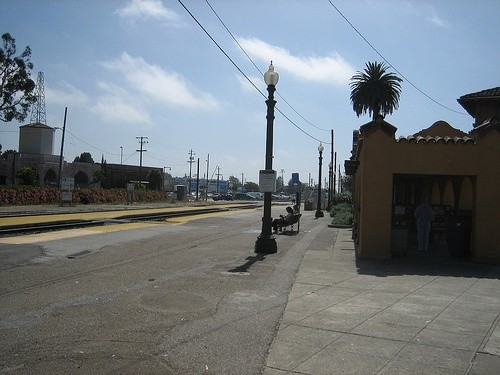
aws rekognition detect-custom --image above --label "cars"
[208,191,292,202]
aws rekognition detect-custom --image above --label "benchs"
[273,214,302,236]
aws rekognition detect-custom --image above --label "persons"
[414,194,435,251]
[293,205,299,214]
[272,207,293,234]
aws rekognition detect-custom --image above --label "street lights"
[326,161,332,212]
[314,142,324,218]
[253,58,278,254]
